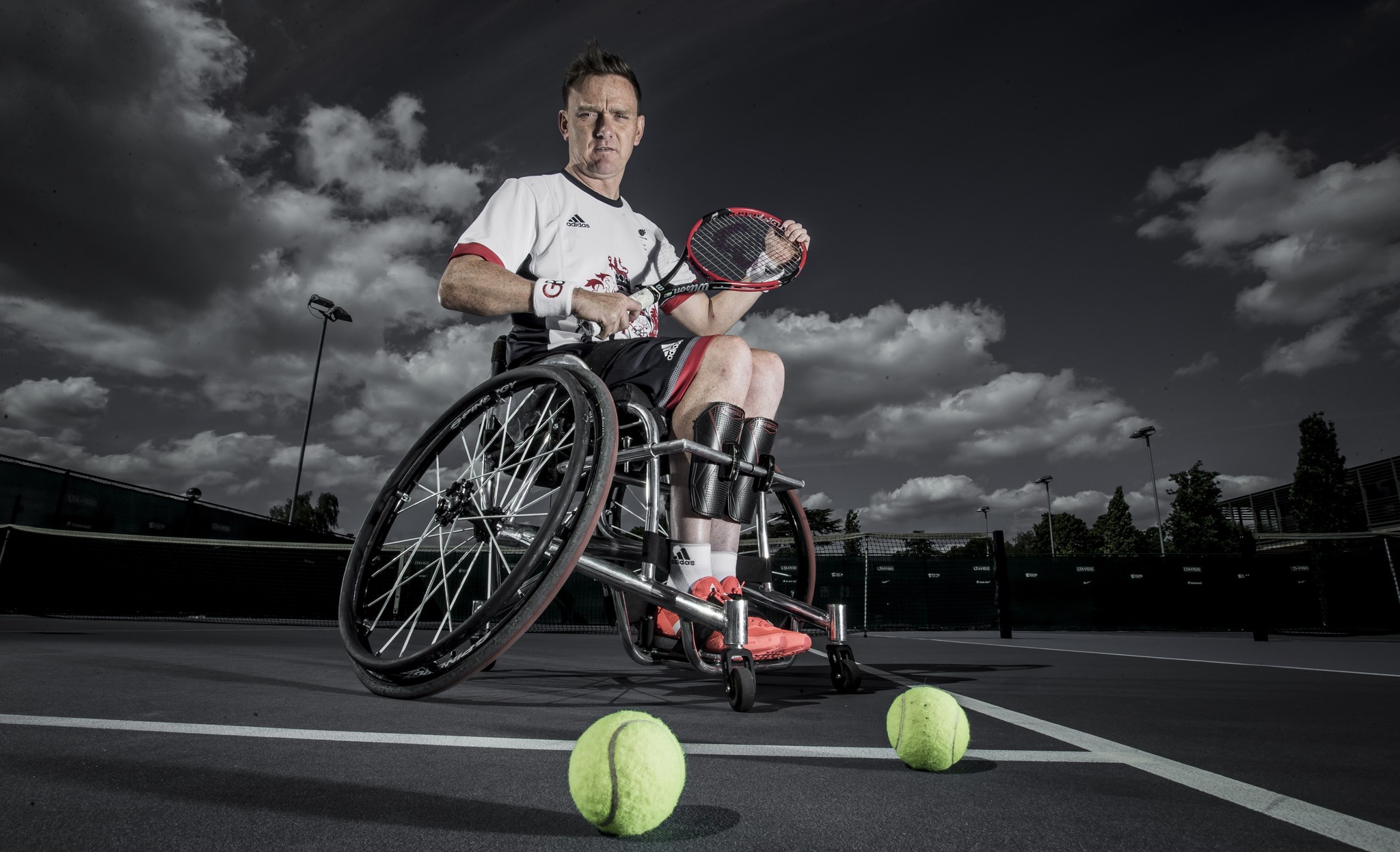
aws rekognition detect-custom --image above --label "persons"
[441,54,815,661]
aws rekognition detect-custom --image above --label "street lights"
[1129,426,1165,557]
[1034,476,1055,555]
[290,294,353,526]
[976,506,990,556]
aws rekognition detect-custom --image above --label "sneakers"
[652,576,812,661]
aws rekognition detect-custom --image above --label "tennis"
[567,707,690,840]
[886,683,973,773]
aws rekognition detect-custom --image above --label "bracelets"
[745,252,786,291]
[529,276,575,315]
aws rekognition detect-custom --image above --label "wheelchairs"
[338,256,861,711]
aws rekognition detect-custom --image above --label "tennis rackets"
[580,207,809,339]
